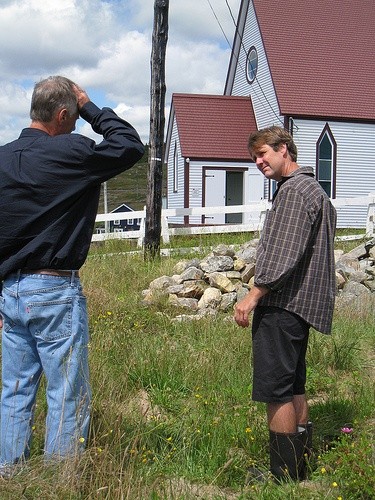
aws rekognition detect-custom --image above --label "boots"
[265,427,307,490]
[298,422,316,465]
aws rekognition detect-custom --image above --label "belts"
[7,267,80,278]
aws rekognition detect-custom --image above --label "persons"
[0,74,148,482]
[235,124,337,486]
